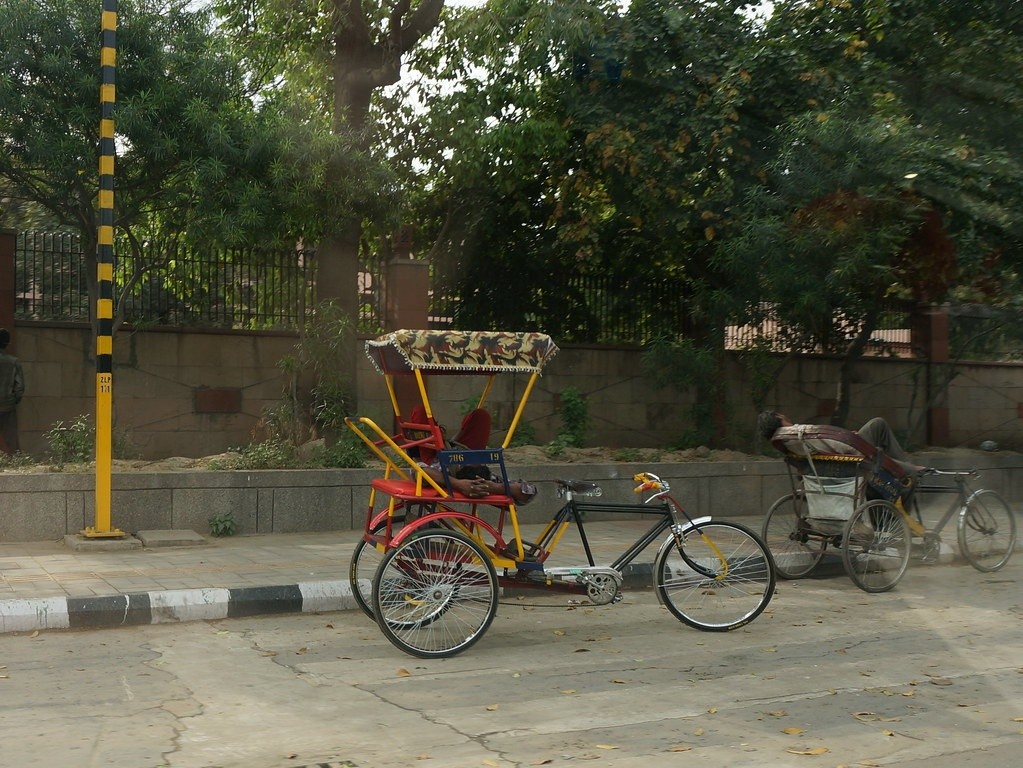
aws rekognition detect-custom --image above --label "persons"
[409,402,537,506]
[0,329,27,456]
[757,406,927,472]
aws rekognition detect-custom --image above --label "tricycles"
[339,329,777,662]
[759,420,1016,595]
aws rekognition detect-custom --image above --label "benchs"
[772,425,912,522]
[344,416,525,569]
